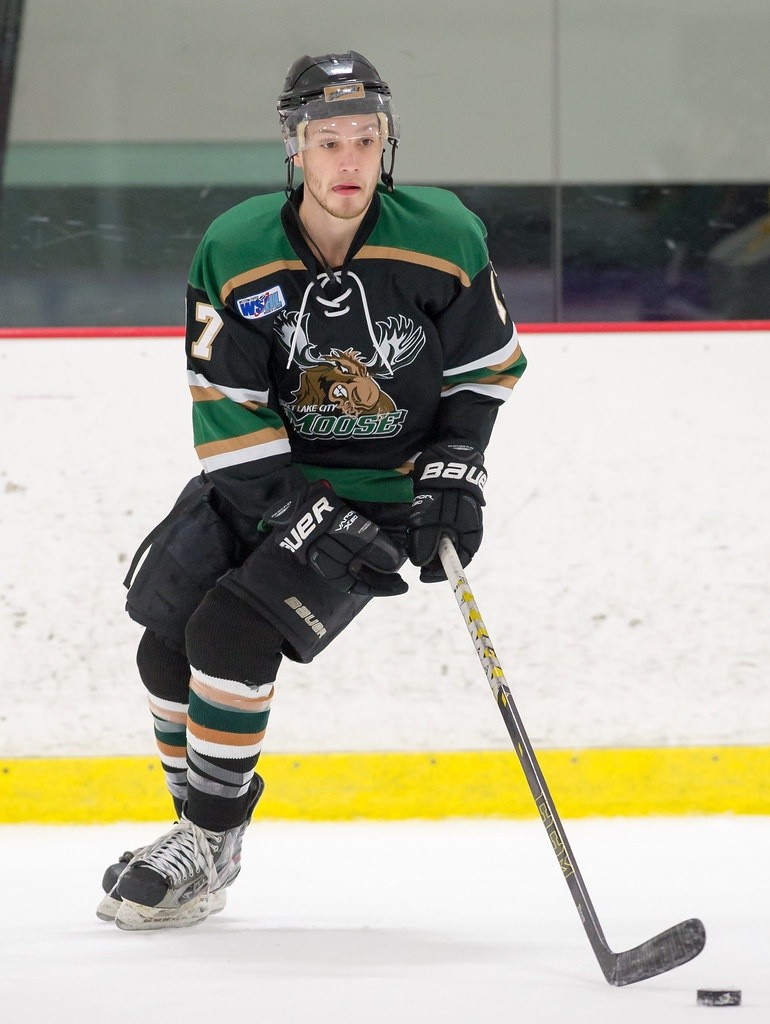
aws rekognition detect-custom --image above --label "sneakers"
[97,771,265,930]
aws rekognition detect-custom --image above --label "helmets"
[276,50,393,125]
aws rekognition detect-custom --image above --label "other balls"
[695,988,743,1008]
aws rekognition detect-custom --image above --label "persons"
[95,50,529,932]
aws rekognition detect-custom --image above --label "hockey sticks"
[437,530,709,988]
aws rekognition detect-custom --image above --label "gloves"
[406,440,487,584]
[257,480,409,597]
